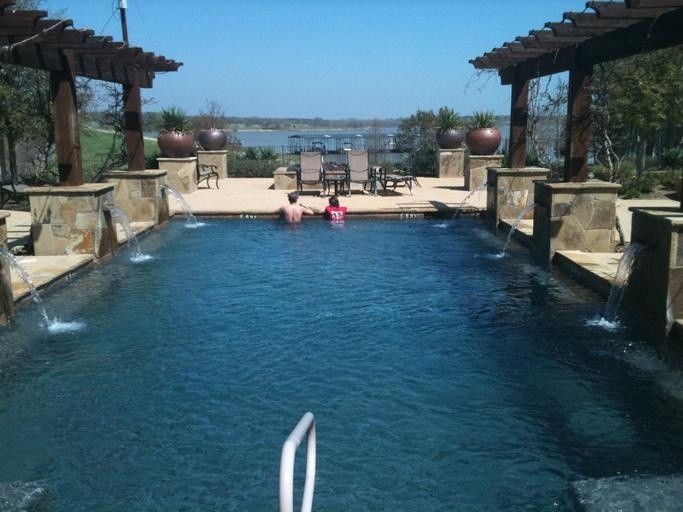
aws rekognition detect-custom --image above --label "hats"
[289,193,298,199]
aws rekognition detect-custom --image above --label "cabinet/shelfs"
[295,150,422,199]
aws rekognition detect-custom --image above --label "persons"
[278,192,314,223]
[325,197,346,221]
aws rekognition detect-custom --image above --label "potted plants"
[435,105,501,156]
[157,98,231,158]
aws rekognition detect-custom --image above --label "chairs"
[0,177,32,210]
[196,165,220,190]
